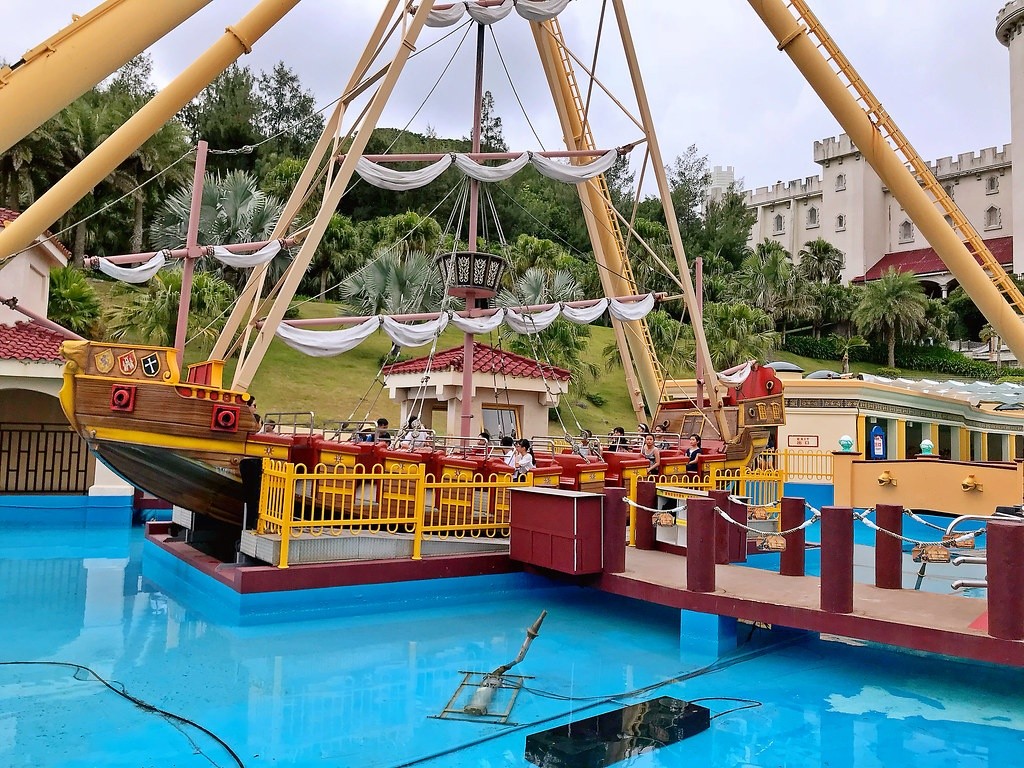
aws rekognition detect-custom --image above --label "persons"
[684,434,704,471]
[359,418,391,447]
[401,416,429,451]
[501,436,536,483]
[608,423,670,452]
[471,432,491,456]
[244,395,278,437]
[572,429,592,456]
[643,434,660,476]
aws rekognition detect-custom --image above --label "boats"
[56,0,792,524]
